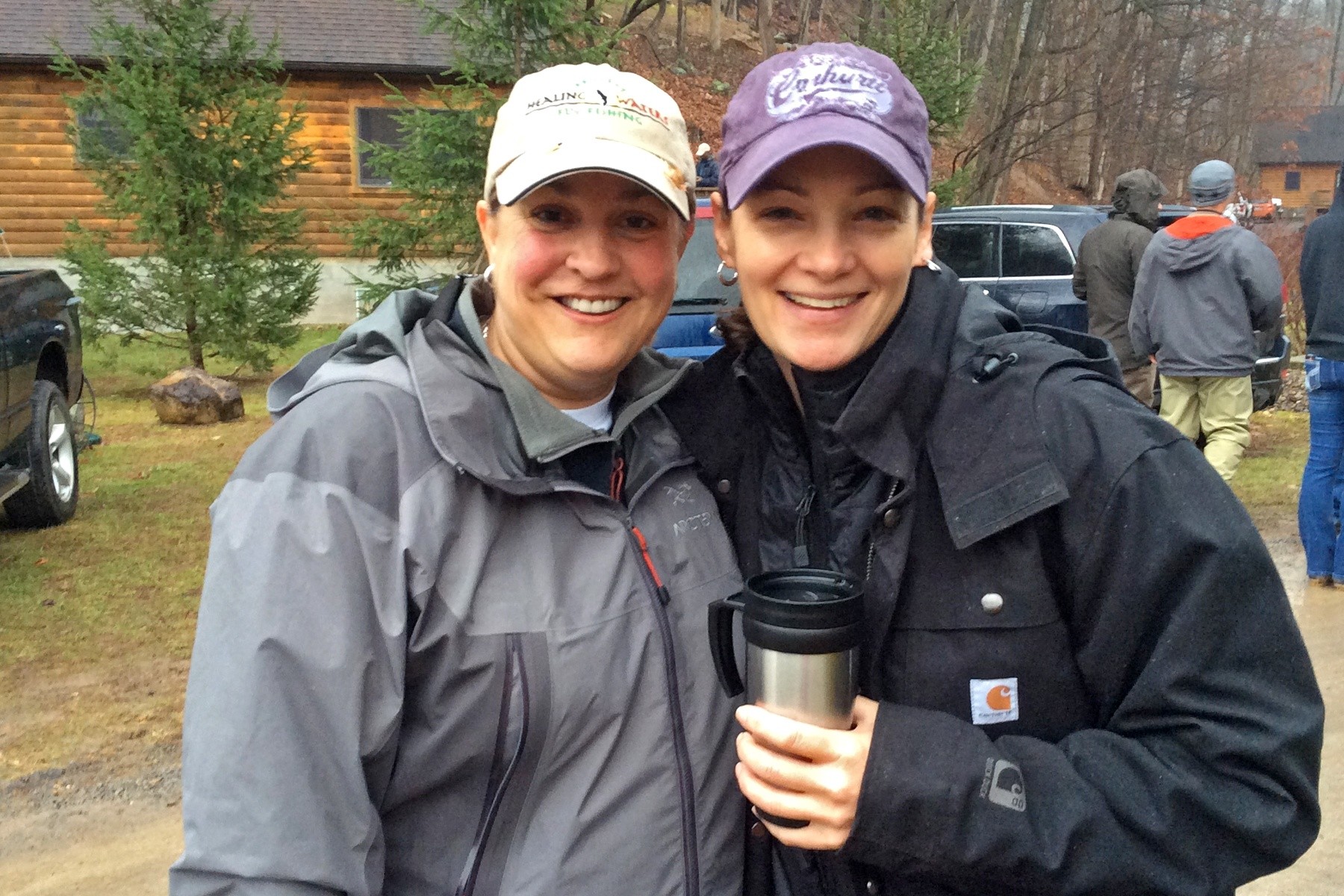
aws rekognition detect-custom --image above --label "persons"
[1130,159,1280,488]
[168,62,753,896]
[673,42,1325,896]
[1073,168,1164,407]
[692,139,717,187]
[1299,169,1344,592]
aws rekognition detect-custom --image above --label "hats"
[483,62,695,223]
[1190,160,1235,207]
[718,42,930,210]
[696,143,710,156]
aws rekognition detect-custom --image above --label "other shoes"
[1309,575,1331,587]
[1333,580,1344,588]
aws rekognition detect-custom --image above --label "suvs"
[648,185,745,363]
[929,200,1293,418]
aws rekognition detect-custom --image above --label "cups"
[706,568,867,829]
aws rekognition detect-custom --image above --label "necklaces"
[481,317,489,339]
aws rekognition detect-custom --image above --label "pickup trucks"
[0,267,86,529]
